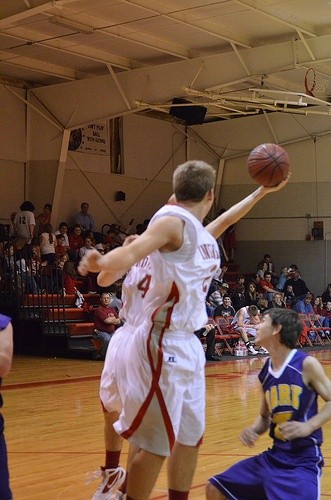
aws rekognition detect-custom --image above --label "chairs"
[204,313,330,355]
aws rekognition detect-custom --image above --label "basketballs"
[248,143,290,187]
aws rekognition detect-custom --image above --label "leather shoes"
[207,356,220,361]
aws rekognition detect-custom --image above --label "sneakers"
[91,466,127,500]
[247,346,269,355]
[87,291,97,295]
[32,288,45,294]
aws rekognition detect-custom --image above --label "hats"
[221,283,229,288]
[304,294,312,299]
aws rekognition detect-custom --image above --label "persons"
[91,192,176,500]
[94,292,121,360]
[204,208,237,263]
[79,219,150,314]
[205,308,331,500]
[38,204,52,231]
[195,301,222,361]
[292,283,331,343]
[9,212,18,240]
[205,254,307,320]
[74,202,94,236]
[0,314,13,500]
[78,160,290,500]
[0,223,95,293]
[231,305,270,355]
[14,201,36,244]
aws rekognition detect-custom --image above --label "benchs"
[22,260,248,349]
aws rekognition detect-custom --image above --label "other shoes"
[96,355,105,361]
